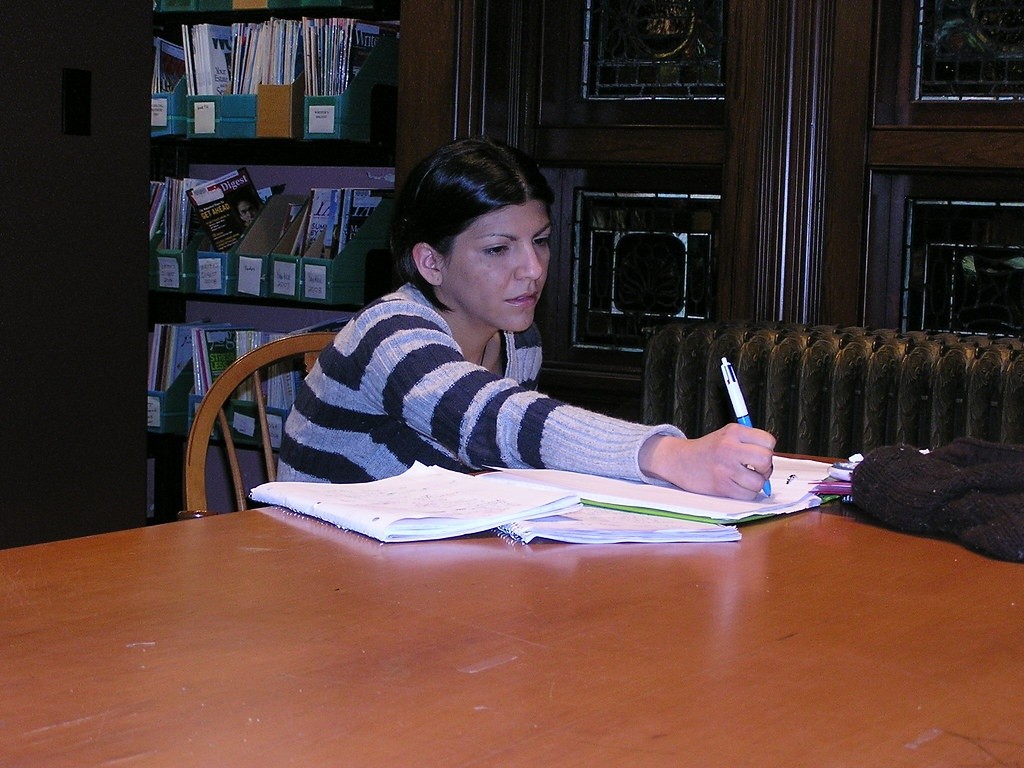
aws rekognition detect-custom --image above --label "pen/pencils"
[717,355,772,499]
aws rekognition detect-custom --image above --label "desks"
[0,451,1024,768]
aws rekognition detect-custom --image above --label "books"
[146,166,394,261]
[249,461,742,546]
[152,16,398,97]
[142,317,348,414]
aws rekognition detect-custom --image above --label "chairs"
[177,332,338,521]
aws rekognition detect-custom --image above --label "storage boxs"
[220,92,257,138]
[268,198,324,301]
[151,221,205,294]
[233,195,307,298]
[187,384,222,441]
[264,403,292,452]
[194,184,288,297]
[225,395,267,446]
[185,94,221,139]
[147,356,194,434]
[150,73,187,136]
[256,72,305,138]
[300,193,398,305]
[303,34,399,141]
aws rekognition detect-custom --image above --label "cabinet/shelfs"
[147,1,403,525]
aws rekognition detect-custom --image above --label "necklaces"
[480,345,486,365]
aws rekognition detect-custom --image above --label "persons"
[275,137,776,500]
[237,194,260,226]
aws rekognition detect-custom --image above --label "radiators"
[640,320,1024,459]
